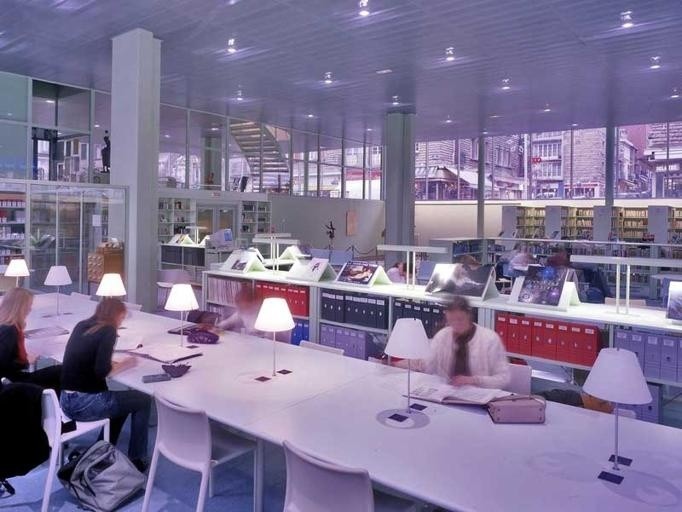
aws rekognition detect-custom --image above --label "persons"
[59,296,151,473]
[413,295,512,389]
[0,286,60,393]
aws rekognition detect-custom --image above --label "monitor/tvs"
[216,229,233,247]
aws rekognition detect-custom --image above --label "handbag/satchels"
[58,440,147,512]
[487,394,545,424]
[187,331,219,344]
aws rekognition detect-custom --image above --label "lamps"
[5,259,30,289]
[95,273,127,296]
[44,265,72,314]
[164,283,199,346]
[254,297,296,376]
[385,317,431,412]
[583,346,653,470]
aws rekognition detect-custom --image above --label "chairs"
[142,393,258,512]
[299,338,345,355]
[505,362,533,396]
[283,441,375,512]
[40,388,110,511]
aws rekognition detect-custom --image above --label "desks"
[23,294,682,512]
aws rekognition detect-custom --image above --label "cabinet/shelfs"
[236,200,273,238]
[200,269,681,389]
[158,198,196,243]
[86,251,123,294]
[432,238,550,267]
[0,201,108,259]
[502,205,682,244]
